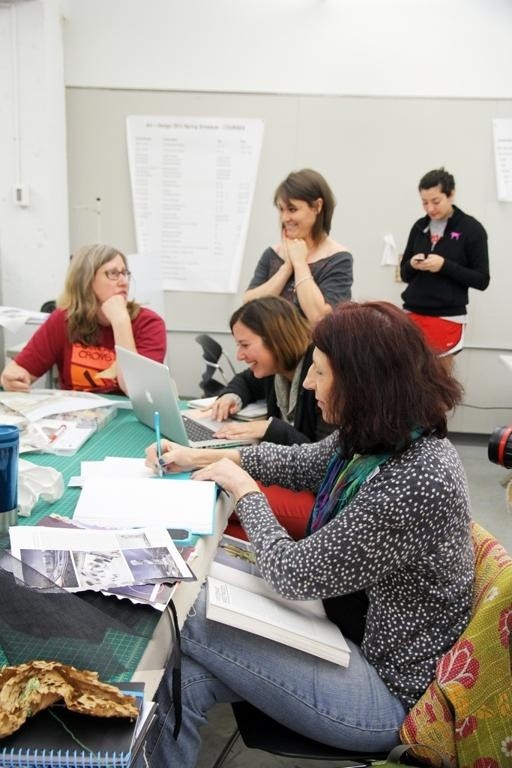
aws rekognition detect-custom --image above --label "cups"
[0,424,20,537]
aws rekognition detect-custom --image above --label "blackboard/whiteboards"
[63,85,512,351]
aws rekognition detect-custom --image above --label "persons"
[397,167,488,376]
[240,168,353,326]
[199,292,339,447]
[133,299,481,768]
[1,242,166,395]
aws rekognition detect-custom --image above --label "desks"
[0,384,235,768]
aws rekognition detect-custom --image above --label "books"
[0,403,120,454]
[190,391,273,424]
[204,535,353,669]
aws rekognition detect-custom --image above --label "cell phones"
[133,526,192,547]
[414,259,425,263]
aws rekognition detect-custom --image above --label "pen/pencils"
[48,425,67,443]
[154,411,163,478]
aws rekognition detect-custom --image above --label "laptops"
[115,345,252,449]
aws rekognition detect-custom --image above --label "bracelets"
[293,272,314,290]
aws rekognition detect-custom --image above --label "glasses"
[93,267,131,281]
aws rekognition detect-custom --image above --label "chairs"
[212,518,512,767]
[197,332,235,392]
